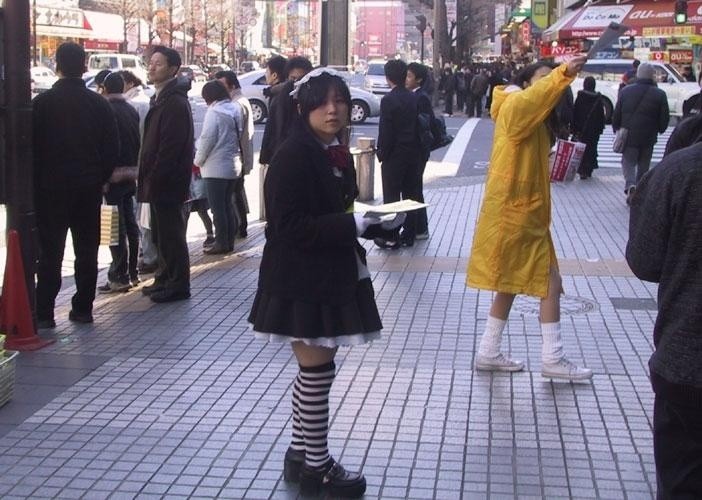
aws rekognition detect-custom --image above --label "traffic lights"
[414,15,425,31]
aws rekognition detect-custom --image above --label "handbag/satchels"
[550,140,587,185]
[99,203,121,246]
[612,128,629,154]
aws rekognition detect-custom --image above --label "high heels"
[284,446,367,499]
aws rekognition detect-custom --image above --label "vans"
[88,53,149,90]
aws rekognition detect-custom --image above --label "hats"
[289,67,349,99]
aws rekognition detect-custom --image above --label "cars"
[364,59,394,94]
[235,67,382,125]
[82,73,101,92]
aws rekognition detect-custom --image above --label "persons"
[610,59,702,207]
[259,56,312,219]
[465,55,594,381]
[378,58,443,246]
[624,140,701,499]
[437,59,525,119]
[544,62,606,180]
[248,66,406,499]
[33,41,254,328]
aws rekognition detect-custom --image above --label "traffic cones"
[1,227,58,353]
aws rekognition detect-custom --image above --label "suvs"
[208,63,231,82]
[568,57,702,124]
[177,64,208,83]
[239,61,260,74]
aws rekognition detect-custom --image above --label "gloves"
[355,212,380,237]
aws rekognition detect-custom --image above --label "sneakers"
[475,353,524,372]
[96,275,140,293]
[68,310,93,322]
[542,356,594,380]
[142,282,191,302]
[202,237,233,254]
